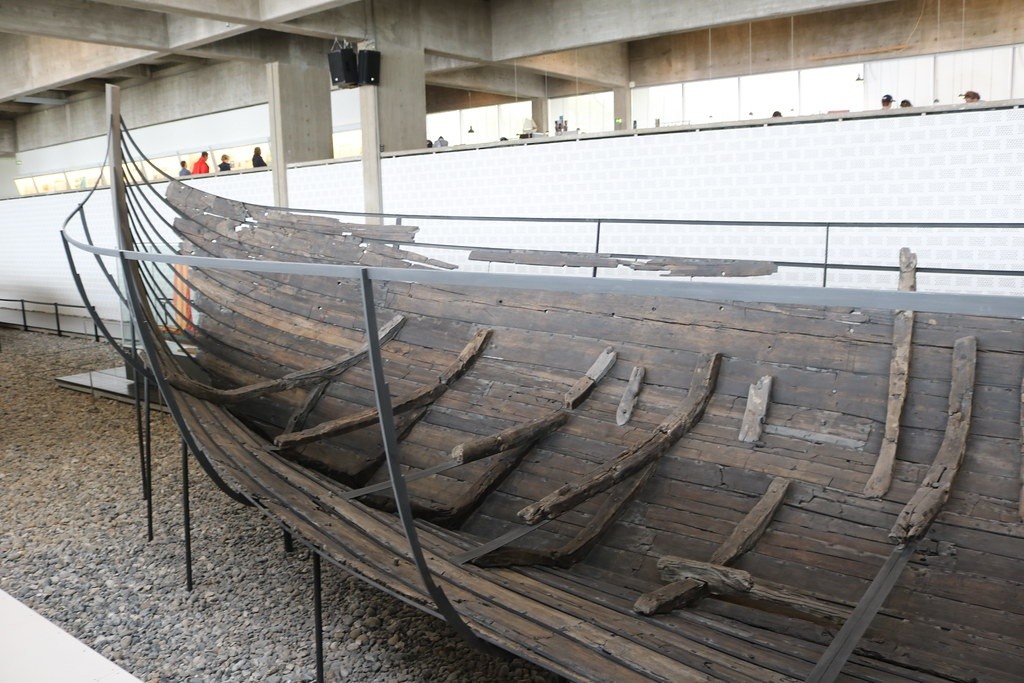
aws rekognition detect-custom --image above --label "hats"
[959,91,980,99]
[882,95,895,102]
[900,100,912,107]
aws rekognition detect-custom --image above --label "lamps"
[467,92,475,133]
[856,52,864,82]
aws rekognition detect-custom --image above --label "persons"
[252,146,267,167]
[963,91,986,103]
[192,151,210,174]
[179,160,191,176]
[772,111,783,117]
[899,99,912,107]
[880,94,896,110]
[217,155,231,171]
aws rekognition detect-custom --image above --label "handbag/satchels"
[523,118,537,132]
[434,136,447,148]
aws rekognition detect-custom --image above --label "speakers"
[357,50,380,85]
[328,48,357,85]
